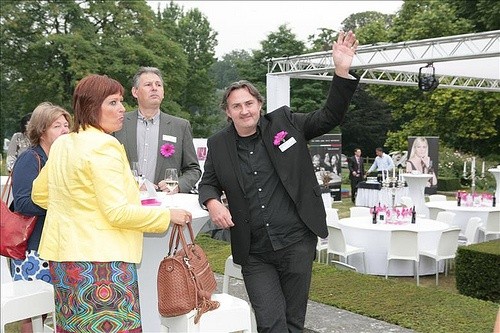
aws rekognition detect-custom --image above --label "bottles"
[457,192,462,206]
[492,192,497,208]
[411,206,416,224]
[372,206,377,224]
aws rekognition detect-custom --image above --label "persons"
[7,112,32,173]
[198,31,359,333]
[365,146,394,182]
[406,137,431,175]
[312,152,341,176]
[349,148,366,202]
[10,102,73,333]
[113,67,202,194]
[32,73,192,333]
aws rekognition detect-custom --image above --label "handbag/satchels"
[0,149,41,260]
[157,223,220,324]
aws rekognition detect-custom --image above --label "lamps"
[419,62,440,93]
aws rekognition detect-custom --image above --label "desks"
[441,201,500,246]
[136,193,210,323]
[355,189,412,208]
[340,218,452,276]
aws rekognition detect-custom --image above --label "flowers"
[274,131,291,150]
[161,143,175,158]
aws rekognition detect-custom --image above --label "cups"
[165,168,178,195]
[130,162,140,186]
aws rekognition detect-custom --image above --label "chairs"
[317,194,500,287]
[195,256,258,333]
